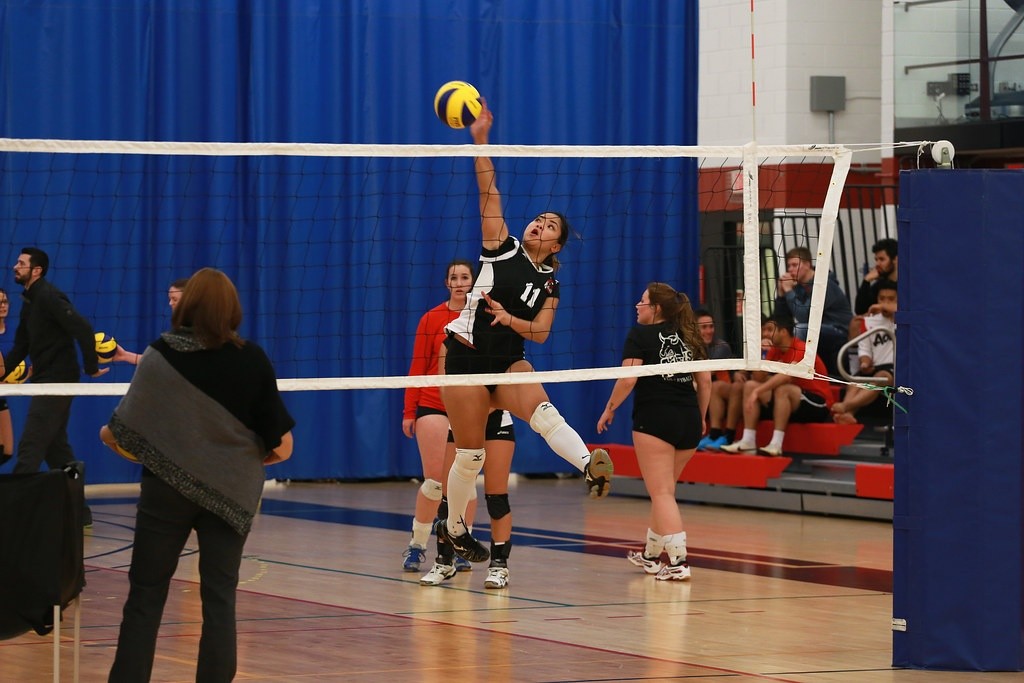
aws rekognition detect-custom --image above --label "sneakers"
[420,559,456,585]
[655,561,691,581]
[627,550,661,574]
[401,544,427,572]
[582,448,614,500]
[454,554,473,571]
[433,515,490,562]
[485,567,510,588]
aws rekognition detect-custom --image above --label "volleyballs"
[95,332,117,364]
[5,360,28,383]
[433,80,483,130]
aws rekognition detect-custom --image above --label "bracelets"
[135,354,139,365]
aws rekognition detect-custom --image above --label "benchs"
[587,314,893,521]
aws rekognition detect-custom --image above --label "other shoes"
[706,436,732,451]
[697,435,714,450]
[760,445,782,456]
[720,442,757,454]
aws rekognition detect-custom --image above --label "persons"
[112,280,187,366]
[694,306,734,421]
[697,315,834,456]
[401,260,478,571]
[99,267,297,683]
[433,95,613,569]
[0,248,110,529]
[767,246,854,372]
[599,279,711,584]
[829,277,897,427]
[0,287,14,464]
[418,399,514,590]
[845,237,897,375]
